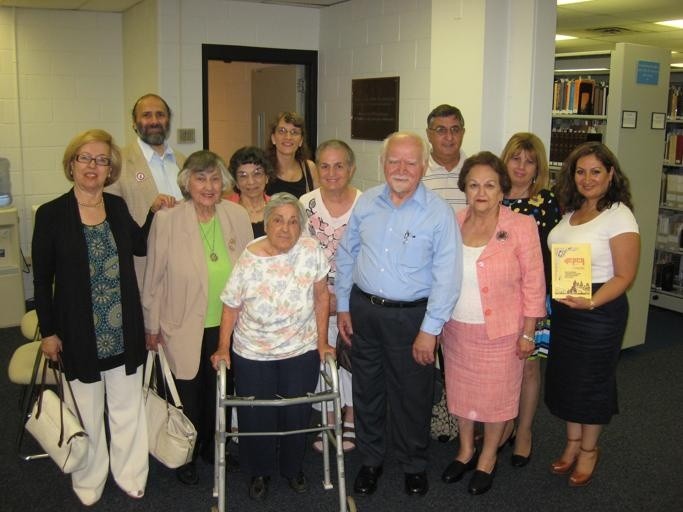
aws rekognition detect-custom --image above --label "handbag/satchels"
[430,345,460,443]
[24,352,90,474]
[140,344,197,468]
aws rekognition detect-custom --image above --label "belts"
[352,283,427,308]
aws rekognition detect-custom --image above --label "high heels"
[550,439,582,476]
[569,447,600,487]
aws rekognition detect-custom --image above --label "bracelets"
[588,298,594,311]
[520,335,535,343]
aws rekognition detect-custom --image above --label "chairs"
[7,338,66,460]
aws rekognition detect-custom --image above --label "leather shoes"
[175,462,198,483]
[292,476,311,494]
[512,442,534,466]
[404,473,428,498]
[355,465,383,495]
[498,426,516,452]
[442,449,477,482]
[469,462,496,495]
[250,475,267,502]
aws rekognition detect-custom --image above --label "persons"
[291,139,367,456]
[335,130,464,500]
[432,152,547,495]
[223,145,274,444]
[417,104,478,216]
[539,141,642,488]
[103,93,191,298]
[29,129,180,507]
[265,111,319,203]
[208,191,337,502]
[496,132,564,467]
[140,150,254,489]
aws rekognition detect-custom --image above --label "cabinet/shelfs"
[546,41,670,354]
[649,66,683,317]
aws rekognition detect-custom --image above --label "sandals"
[312,430,323,453]
[341,425,355,451]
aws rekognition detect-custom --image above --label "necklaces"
[77,198,102,207]
[196,211,218,262]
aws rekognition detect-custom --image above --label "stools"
[20,307,43,341]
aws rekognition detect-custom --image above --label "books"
[549,243,592,301]
[548,76,610,168]
[649,84,682,294]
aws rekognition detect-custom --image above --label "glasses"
[277,126,301,135]
[467,183,497,190]
[428,126,462,134]
[75,153,111,166]
[237,170,266,177]
